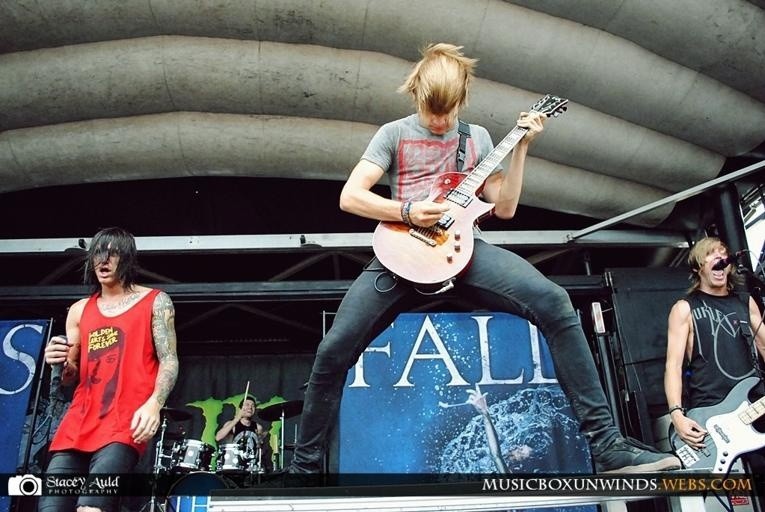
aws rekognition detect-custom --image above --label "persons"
[661,232,765,510]
[212,395,269,467]
[282,42,684,478]
[35,226,183,510]
[79,327,129,419]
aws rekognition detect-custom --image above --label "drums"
[215,444,248,472]
[171,439,215,473]
[164,472,239,512]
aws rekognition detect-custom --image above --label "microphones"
[49,334,67,393]
[712,251,744,271]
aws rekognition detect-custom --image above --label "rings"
[150,428,156,433]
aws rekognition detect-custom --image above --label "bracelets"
[400,199,414,229]
[667,406,682,415]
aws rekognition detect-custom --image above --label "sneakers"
[593,437,681,477]
[245,465,324,487]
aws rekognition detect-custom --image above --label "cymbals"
[258,400,303,421]
[160,408,192,421]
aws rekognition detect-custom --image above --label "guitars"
[668,376,764,475]
[372,94,568,283]
